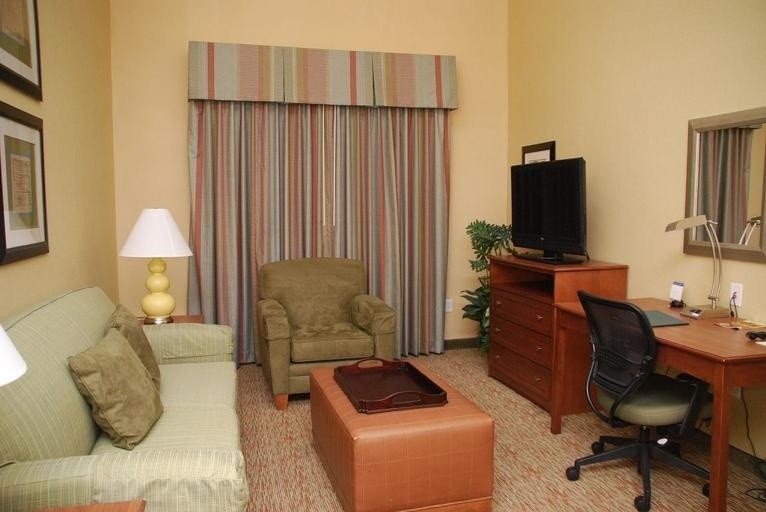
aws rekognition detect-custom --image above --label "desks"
[18,498,147,512]
[548,293,766,512]
[132,314,206,325]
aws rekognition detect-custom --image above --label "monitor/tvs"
[510,156,588,265]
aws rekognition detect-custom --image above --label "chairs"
[564,288,715,511]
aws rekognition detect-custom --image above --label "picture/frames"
[0,0,44,103]
[0,98,49,265]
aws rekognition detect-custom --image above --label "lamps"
[116,208,195,325]
[739,216,762,246]
[665,214,731,320]
[0,323,25,389]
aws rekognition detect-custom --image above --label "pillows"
[66,328,165,451]
[108,302,160,393]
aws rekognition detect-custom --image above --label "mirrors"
[682,105,766,264]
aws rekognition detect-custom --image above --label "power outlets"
[730,283,743,307]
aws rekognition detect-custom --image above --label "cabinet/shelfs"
[487,254,628,434]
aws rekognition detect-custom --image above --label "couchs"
[0,285,252,511]
[256,257,397,411]
